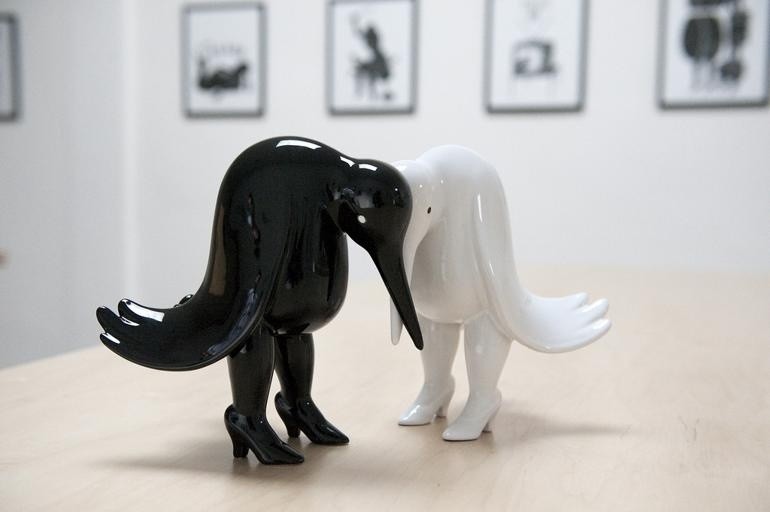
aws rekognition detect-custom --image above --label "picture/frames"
[654,0,768,114]
[321,1,423,118]
[481,0,586,118]
[176,0,268,119]
[0,10,22,120]
